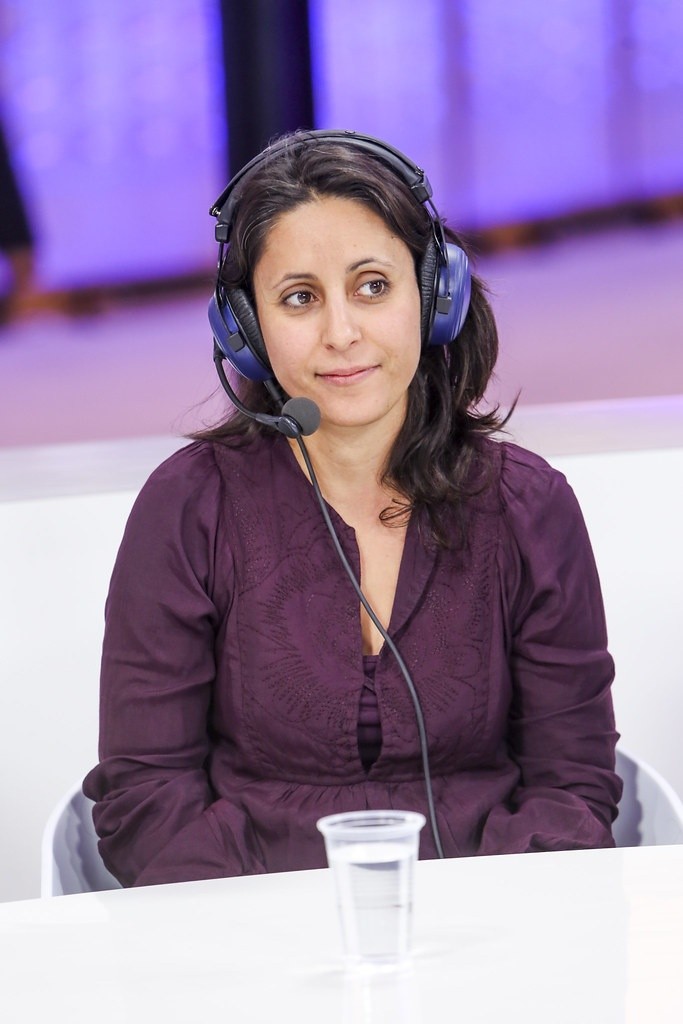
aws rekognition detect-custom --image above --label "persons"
[0,125,37,325]
[79,130,624,886]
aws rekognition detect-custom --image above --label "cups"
[316,810,425,955]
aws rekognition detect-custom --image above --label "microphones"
[210,348,321,440]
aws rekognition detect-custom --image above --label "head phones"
[206,130,472,382]
